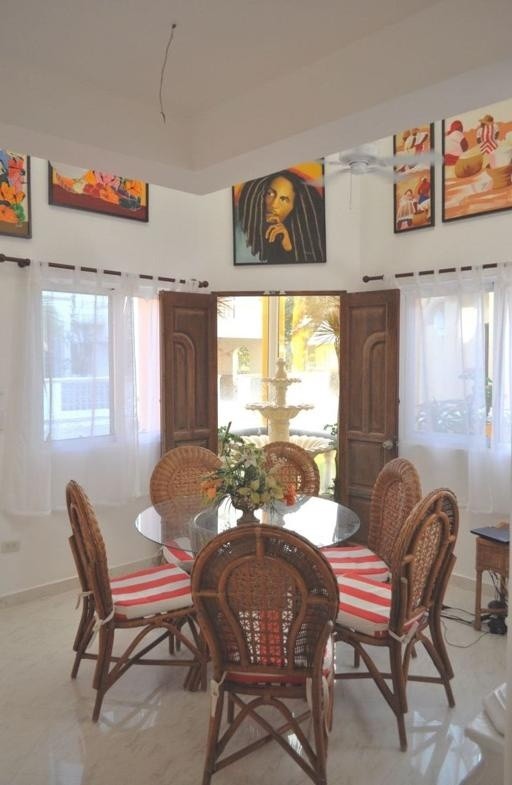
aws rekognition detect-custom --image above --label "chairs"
[149,444,231,652]
[333,487,459,752]
[257,443,319,498]
[65,480,208,724]
[318,457,421,667]
[190,523,340,785]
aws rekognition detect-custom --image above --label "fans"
[297,142,444,190]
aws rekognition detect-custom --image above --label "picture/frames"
[231,157,328,265]
[47,160,150,224]
[393,97,512,234]
[0,147,33,239]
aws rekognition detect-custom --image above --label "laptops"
[470,526,510,546]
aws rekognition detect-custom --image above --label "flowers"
[198,419,299,524]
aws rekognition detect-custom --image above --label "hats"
[478,115,494,125]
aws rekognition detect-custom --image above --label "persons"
[416,175,430,210]
[397,130,410,150]
[444,120,468,167]
[474,114,498,157]
[398,128,428,172]
[236,168,325,266]
[395,189,415,231]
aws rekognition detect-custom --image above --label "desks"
[473,522,509,632]
[135,489,362,552]
